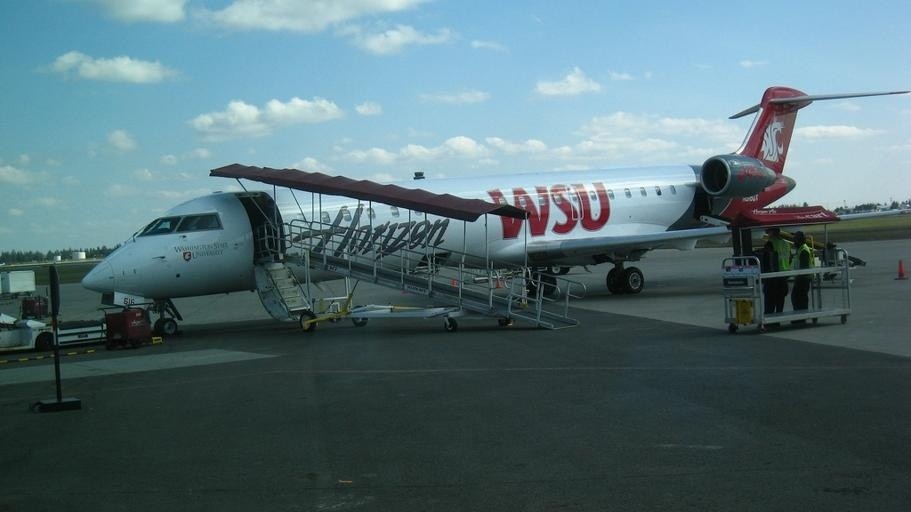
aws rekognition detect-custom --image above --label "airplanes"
[80,86,911,338]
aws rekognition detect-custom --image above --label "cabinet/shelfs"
[720,248,852,334]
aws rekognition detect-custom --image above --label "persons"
[787,231,818,325]
[757,227,794,334]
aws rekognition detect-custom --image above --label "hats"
[765,227,780,233]
[791,231,805,237]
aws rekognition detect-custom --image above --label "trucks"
[1,270,36,298]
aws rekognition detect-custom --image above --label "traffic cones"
[895,260,908,281]
